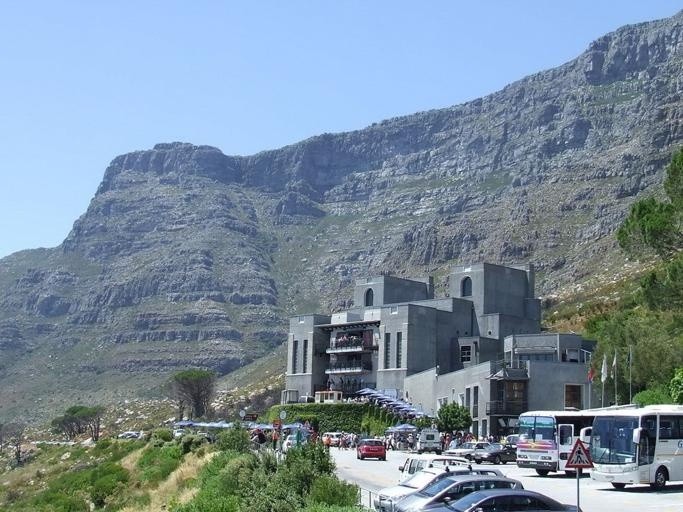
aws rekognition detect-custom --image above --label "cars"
[172,429,214,444]
[374,441,582,512]
[281,434,306,454]
[356,439,386,460]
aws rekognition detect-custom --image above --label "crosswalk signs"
[565,439,595,468]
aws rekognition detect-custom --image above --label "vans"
[503,434,518,452]
[416,429,442,455]
[322,432,349,446]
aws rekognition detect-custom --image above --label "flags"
[625,350,631,369]
[587,363,595,382]
[600,355,607,383]
[610,351,616,380]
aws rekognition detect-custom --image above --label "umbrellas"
[173,421,309,436]
[354,386,434,433]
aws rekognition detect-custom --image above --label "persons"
[248,427,496,455]
[334,332,363,347]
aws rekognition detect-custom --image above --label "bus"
[516,410,596,475]
[581,404,682,489]
